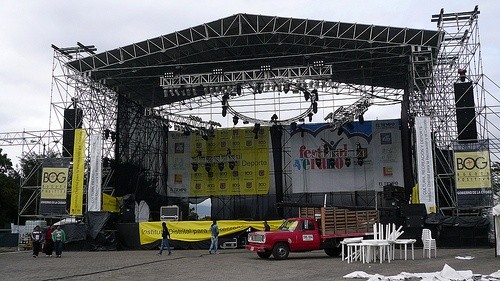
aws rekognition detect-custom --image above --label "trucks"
[247,207,382,260]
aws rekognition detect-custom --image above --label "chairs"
[421,229,436,259]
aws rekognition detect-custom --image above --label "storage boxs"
[160,206,179,221]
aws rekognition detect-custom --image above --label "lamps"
[205,163,211,172]
[358,158,363,166]
[105,129,116,141]
[315,159,321,167]
[329,159,335,168]
[222,83,318,139]
[160,120,215,141]
[218,163,224,171]
[192,163,198,171]
[103,157,109,167]
[345,158,351,167]
[458,69,466,82]
[229,162,235,170]
[164,86,219,97]
[71,97,77,108]
[337,115,364,136]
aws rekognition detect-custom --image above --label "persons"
[52,226,66,258]
[157,222,172,256]
[263,221,270,231]
[45,223,55,257]
[31,225,43,258]
[209,220,219,254]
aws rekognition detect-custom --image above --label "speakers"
[62,110,83,161]
[377,185,428,227]
[122,199,135,223]
[454,81,478,143]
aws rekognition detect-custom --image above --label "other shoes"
[168,251,171,255]
[157,251,162,254]
[46,254,52,257]
[32,254,39,258]
[56,254,61,258]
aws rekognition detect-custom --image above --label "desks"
[340,223,417,264]
